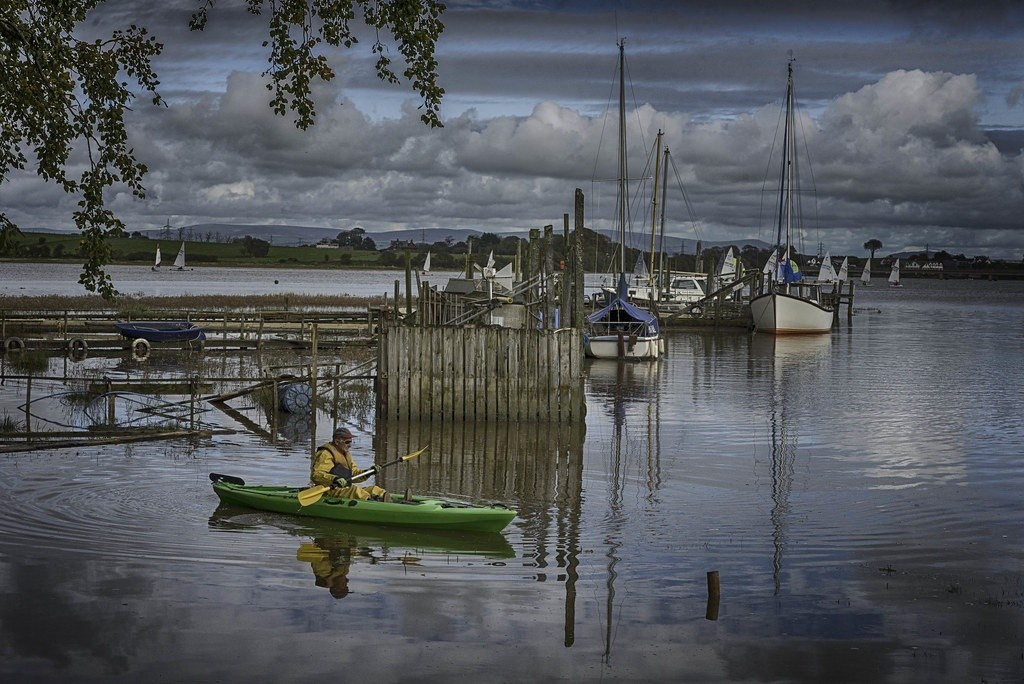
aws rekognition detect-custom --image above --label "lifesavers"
[68,350,88,363]
[4,336,26,350]
[131,337,151,348]
[131,349,151,362]
[690,305,704,319]
[67,337,88,348]
[4,352,24,365]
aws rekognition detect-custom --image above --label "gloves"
[335,478,347,488]
[365,465,382,477]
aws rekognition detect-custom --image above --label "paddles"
[295,543,423,566]
[297,444,430,508]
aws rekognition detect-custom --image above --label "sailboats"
[150,243,165,270]
[721,247,738,284]
[816,252,841,284]
[859,258,875,289]
[170,242,191,271]
[584,70,664,361]
[837,258,850,284]
[752,69,834,334]
[762,249,787,287]
[887,259,903,287]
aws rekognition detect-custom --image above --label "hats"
[333,427,358,439]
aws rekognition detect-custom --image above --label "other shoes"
[404,488,412,501]
[383,492,391,502]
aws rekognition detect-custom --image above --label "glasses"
[339,440,352,444]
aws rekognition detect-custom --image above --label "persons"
[310,427,412,503]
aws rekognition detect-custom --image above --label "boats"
[209,474,518,534]
[602,278,708,318]
[113,321,205,346]
